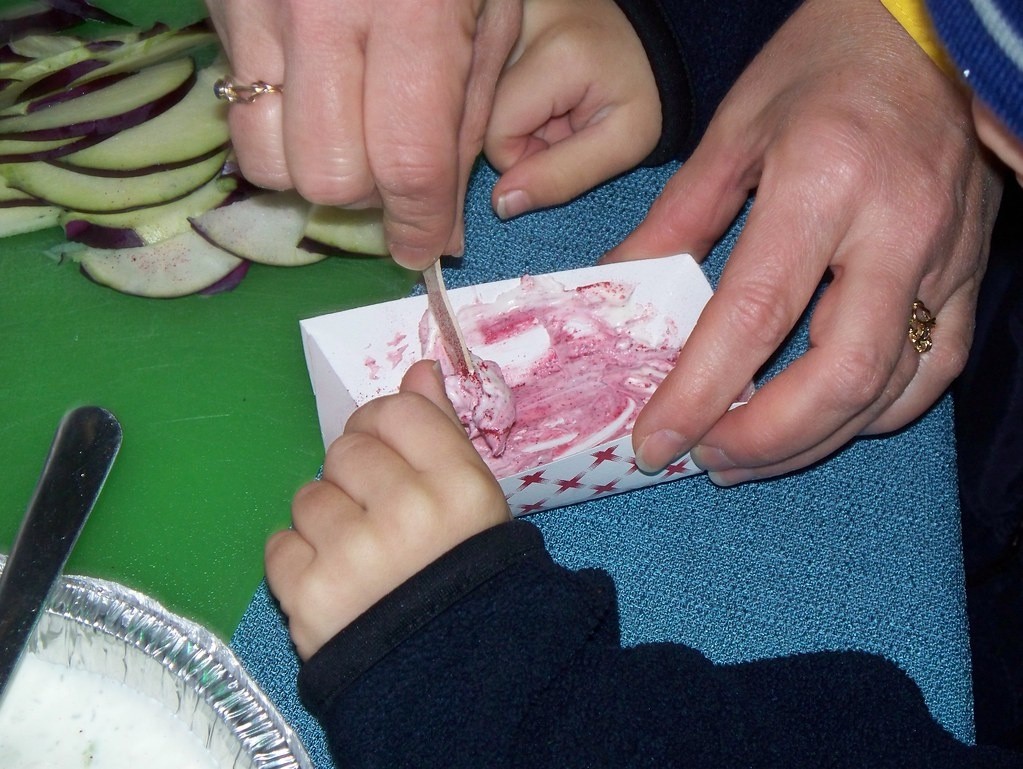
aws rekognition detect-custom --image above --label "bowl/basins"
[0,551,314,769]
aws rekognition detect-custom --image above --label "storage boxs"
[299,253,755,515]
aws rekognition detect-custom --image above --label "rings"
[212,77,284,103]
[909,300,937,351]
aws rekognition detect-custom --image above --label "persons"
[202,0,1023,490]
[263,359,1012,769]
